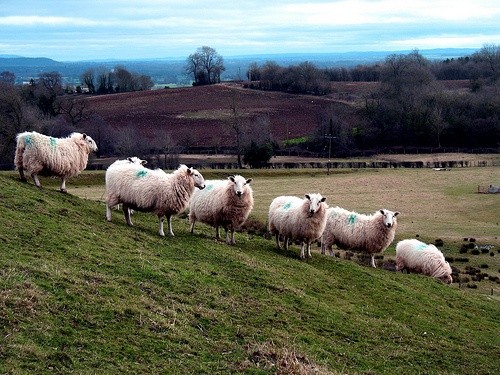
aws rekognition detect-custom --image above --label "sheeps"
[187,174,254,245]
[104,156,206,237]
[13,131,98,193]
[321,206,399,269]
[394,239,452,284]
[268,193,329,260]
